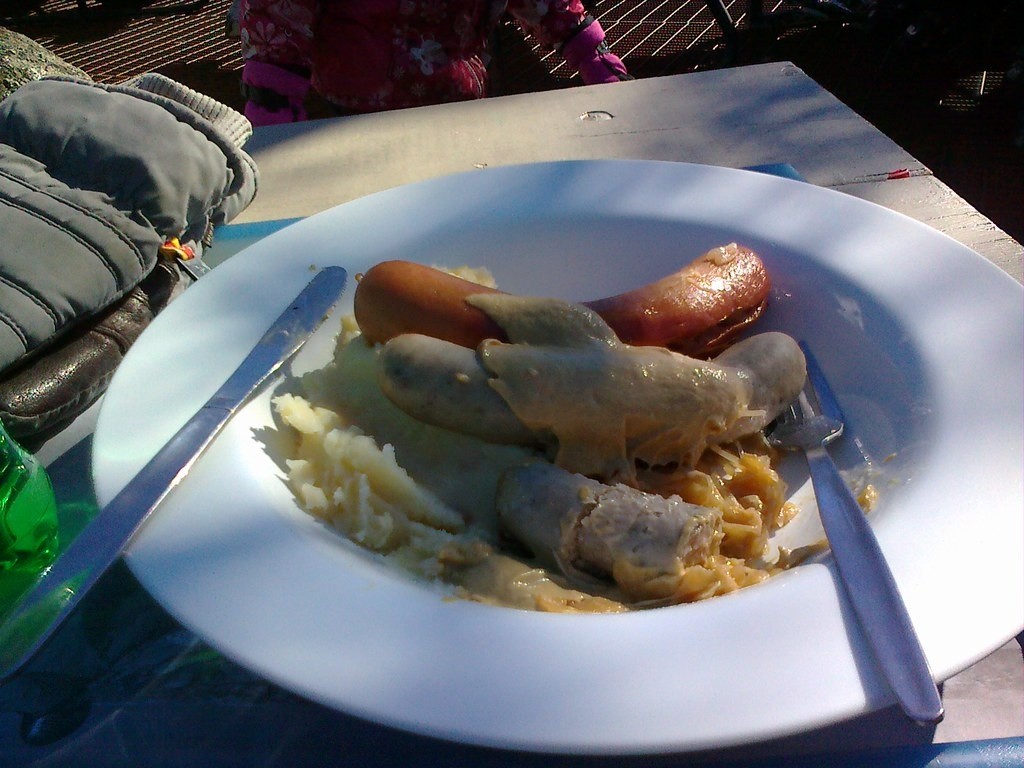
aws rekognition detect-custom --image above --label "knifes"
[0,263,347,680]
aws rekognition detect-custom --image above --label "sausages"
[379,331,808,448]
[352,246,771,363]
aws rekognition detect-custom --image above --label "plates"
[90,158,1023,757]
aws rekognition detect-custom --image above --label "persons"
[224,0,635,126]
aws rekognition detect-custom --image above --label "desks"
[0,59,1024,768]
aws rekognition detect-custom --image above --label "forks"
[761,339,945,730]
